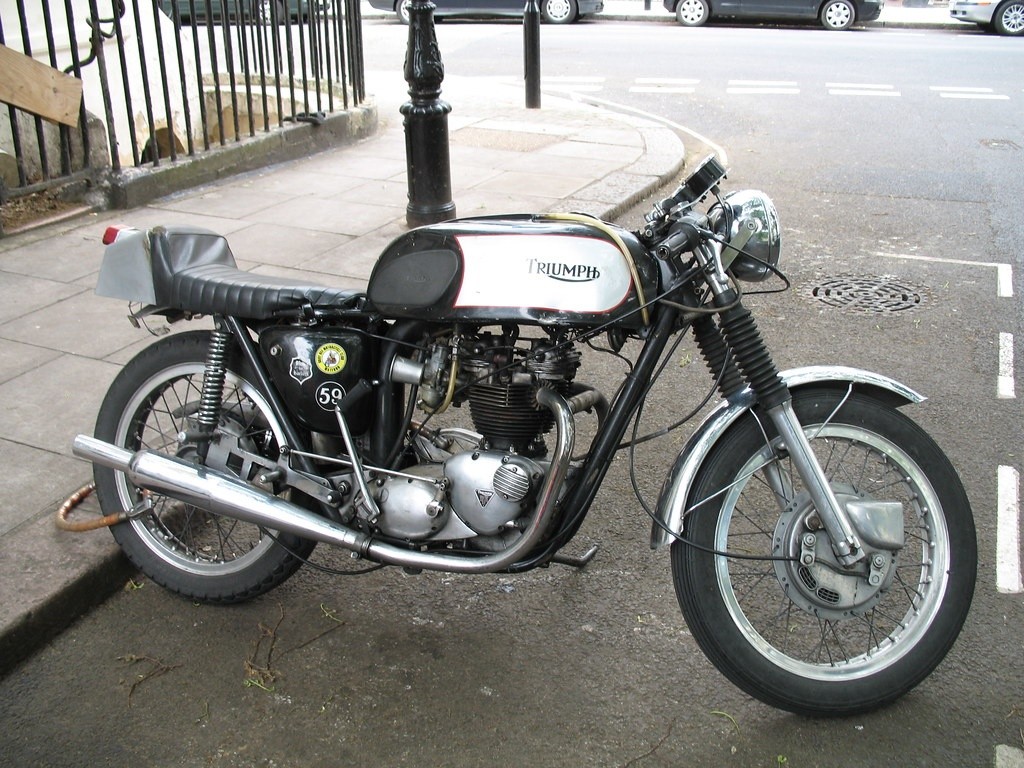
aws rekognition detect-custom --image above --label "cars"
[369,0,605,25]
[948,0,1024,38]
[663,0,884,31]
[155,0,331,27]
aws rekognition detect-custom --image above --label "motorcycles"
[70,153,981,722]
